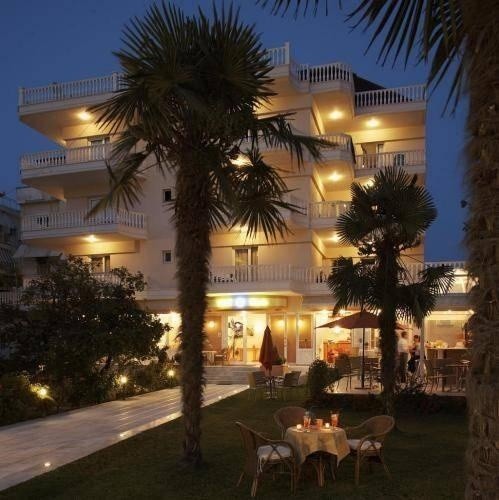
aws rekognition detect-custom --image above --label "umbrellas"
[314,308,409,387]
[259,327,285,396]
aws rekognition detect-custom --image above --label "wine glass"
[331,414,338,431]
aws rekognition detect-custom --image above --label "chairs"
[344,415,395,485]
[215,349,227,366]
[235,421,296,498]
[274,406,319,479]
[341,351,471,394]
[244,369,300,401]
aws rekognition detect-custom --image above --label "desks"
[202,351,218,365]
[236,346,258,361]
[288,426,350,484]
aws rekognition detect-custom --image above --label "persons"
[397,331,410,383]
[408,336,423,373]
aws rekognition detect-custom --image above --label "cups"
[316,419,323,430]
[303,416,311,430]
